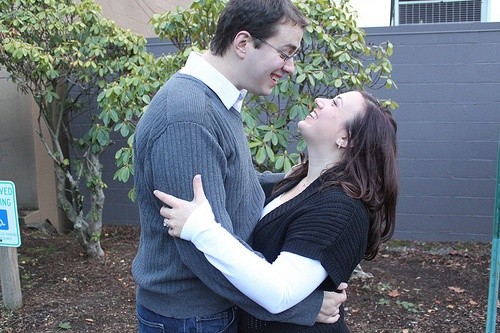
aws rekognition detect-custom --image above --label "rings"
[164,219,168,226]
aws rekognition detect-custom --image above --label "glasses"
[252,32,299,62]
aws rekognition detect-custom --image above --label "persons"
[152,88,398,333]
[131,0,346,333]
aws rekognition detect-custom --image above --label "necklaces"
[302,183,306,186]
